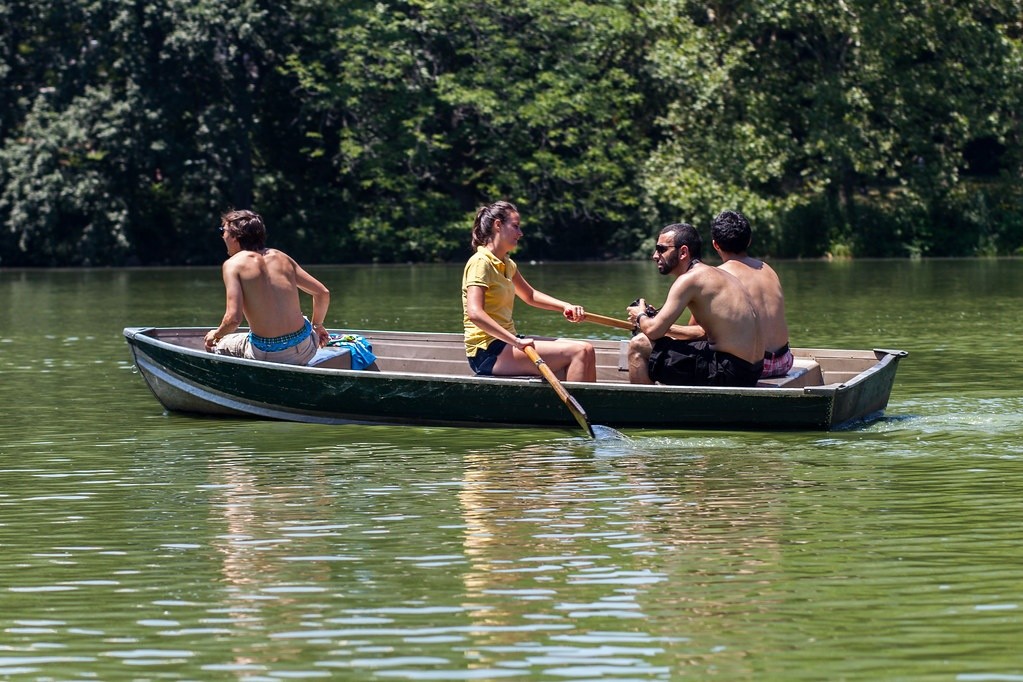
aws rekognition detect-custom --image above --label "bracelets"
[311,321,319,329]
[514,338,522,348]
[636,313,648,329]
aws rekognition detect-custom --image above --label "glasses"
[218,227,231,237]
[655,245,676,255]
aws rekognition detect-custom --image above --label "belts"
[764,342,789,360]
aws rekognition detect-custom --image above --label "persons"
[628,223,764,386]
[687,209,795,378]
[203,210,331,367]
[461,199,598,382]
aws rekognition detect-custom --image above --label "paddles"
[565,308,635,331]
[514,334,596,439]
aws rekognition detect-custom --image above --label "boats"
[121,327,908,433]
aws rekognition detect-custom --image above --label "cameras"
[629,299,659,336]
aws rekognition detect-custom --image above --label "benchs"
[305,346,351,370]
[759,361,822,388]
[473,372,543,380]
